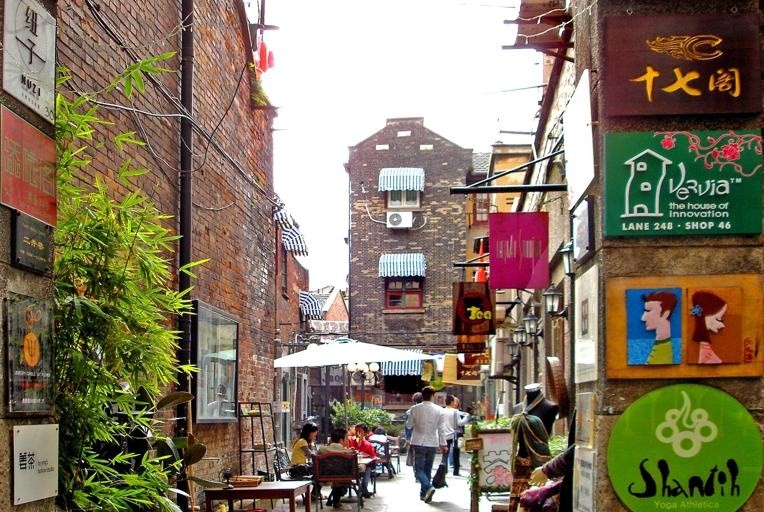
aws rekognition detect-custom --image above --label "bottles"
[327,437,331,446]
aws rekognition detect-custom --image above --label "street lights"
[346,361,383,410]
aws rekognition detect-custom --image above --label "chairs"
[203,423,405,511]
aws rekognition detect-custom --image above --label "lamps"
[512,326,532,349]
[557,240,577,279]
[506,340,519,357]
[544,283,567,319]
[524,309,544,340]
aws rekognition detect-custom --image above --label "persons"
[405,386,449,503]
[206,383,234,418]
[442,394,469,476]
[449,397,476,469]
[317,428,354,510]
[639,291,678,365]
[405,391,424,483]
[348,423,388,498]
[290,421,322,500]
[688,290,728,364]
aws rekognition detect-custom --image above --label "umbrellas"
[273,337,438,449]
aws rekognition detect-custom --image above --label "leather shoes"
[326,501,342,508]
[360,492,372,498]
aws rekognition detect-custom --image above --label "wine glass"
[222,467,232,488]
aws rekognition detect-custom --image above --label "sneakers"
[424,488,435,503]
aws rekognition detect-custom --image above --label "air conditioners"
[385,211,414,229]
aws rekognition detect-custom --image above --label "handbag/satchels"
[432,465,448,488]
[289,464,312,478]
[453,433,465,449]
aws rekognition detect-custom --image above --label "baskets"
[545,356,566,406]
